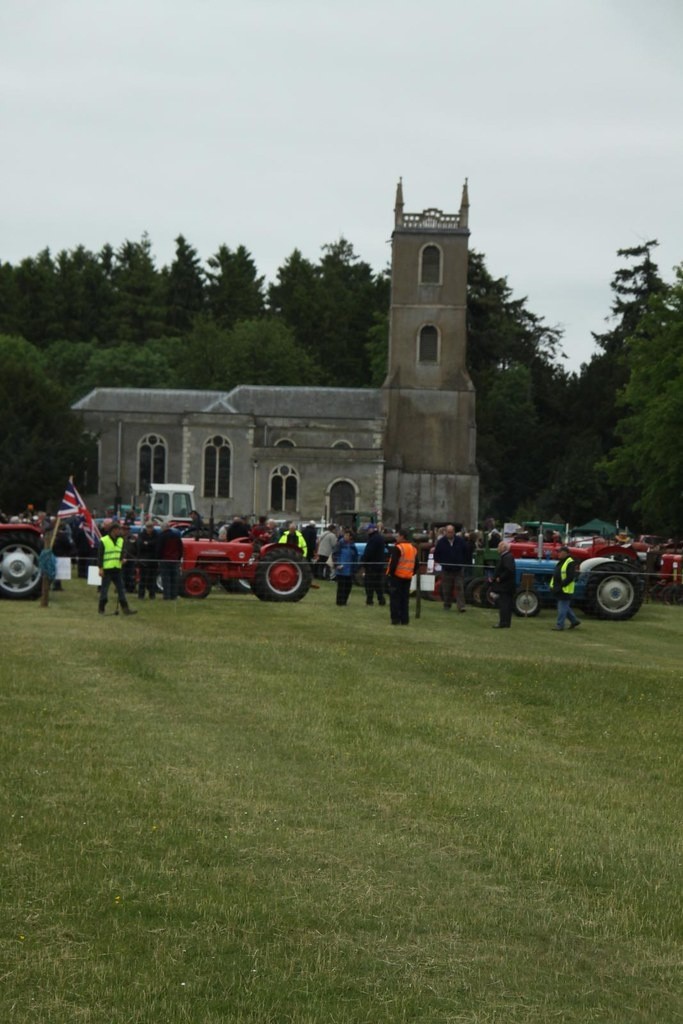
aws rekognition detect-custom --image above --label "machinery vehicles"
[0,514,46,602]
[92,483,199,538]
[154,531,313,603]
[351,520,682,620]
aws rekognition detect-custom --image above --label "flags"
[58,483,103,550]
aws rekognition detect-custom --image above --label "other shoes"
[492,623,510,629]
[459,605,466,612]
[122,608,138,614]
[98,610,104,615]
[444,604,451,609]
[568,621,580,628]
[552,625,563,631]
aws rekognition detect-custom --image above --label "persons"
[0,498,683,634]
